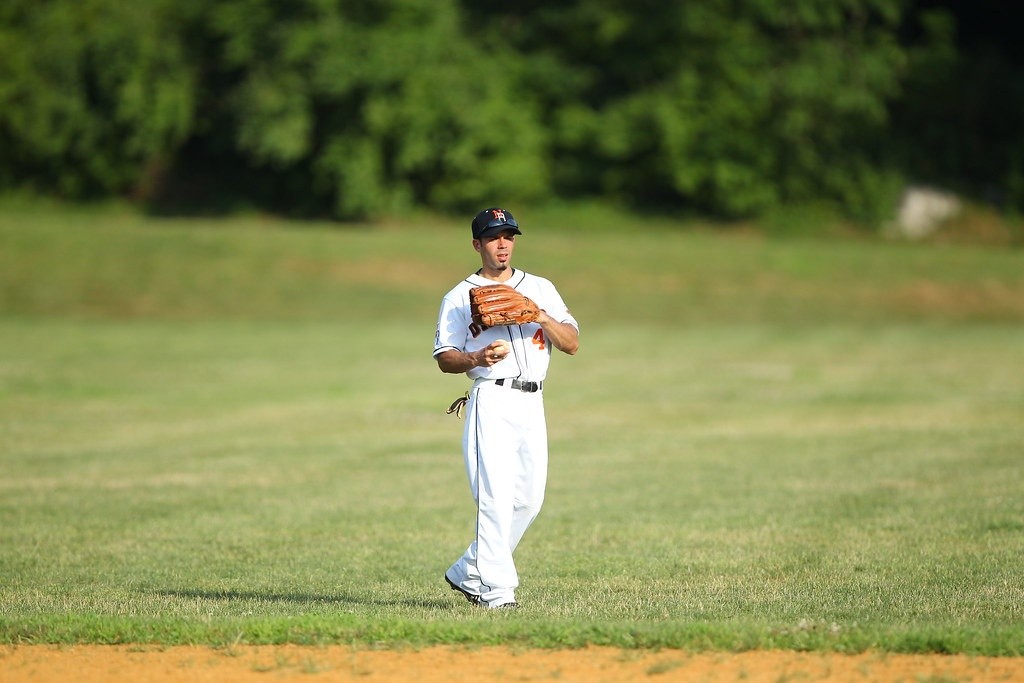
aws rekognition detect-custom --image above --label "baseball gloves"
[469,285,539,328]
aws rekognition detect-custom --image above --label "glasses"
[475,218,519,239]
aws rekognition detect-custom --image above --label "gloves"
[446,390,470,420]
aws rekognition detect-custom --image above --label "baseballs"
[493,338,511,355]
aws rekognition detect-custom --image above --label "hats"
[472,207,522,240]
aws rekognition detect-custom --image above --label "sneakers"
[445,573,489,607]
[497,603,521,609]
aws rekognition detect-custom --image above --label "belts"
[495,379,542,392]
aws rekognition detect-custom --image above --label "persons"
[432,208,579,609]
